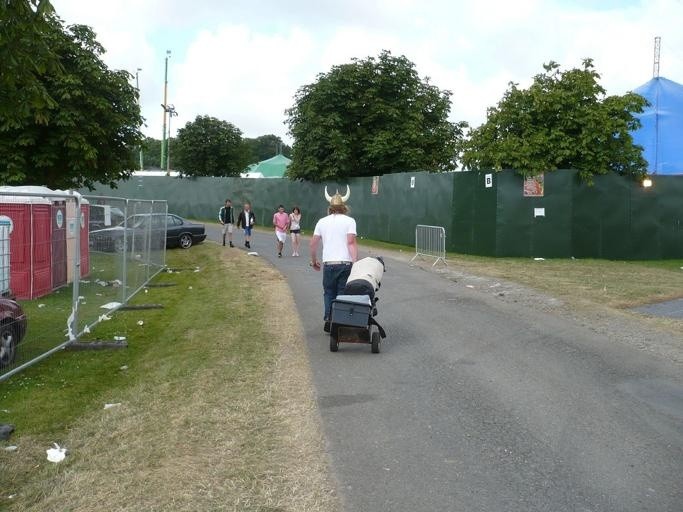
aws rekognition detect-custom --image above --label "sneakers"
[278,252,282,257]
[244,241,250,248]
[222,241,234,247]
[292,252,299,257]
[324,321,330,332]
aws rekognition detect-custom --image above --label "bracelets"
[307,259,317,267]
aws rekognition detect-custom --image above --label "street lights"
[159,50,173,170]
[160,103,178,176]
[134,67,144,171]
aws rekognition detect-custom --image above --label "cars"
[0,294,26,368]
[87,212,207,253]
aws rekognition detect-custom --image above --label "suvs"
[87,204,127,233]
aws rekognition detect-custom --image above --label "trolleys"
[327,267,382,355]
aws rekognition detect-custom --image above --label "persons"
[272,205,288,257]
[218,199,234,247]
[236,202,255,248]
[287,207,301,256]
[309,195,357,333]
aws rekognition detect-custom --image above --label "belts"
[325,261,350,265]
